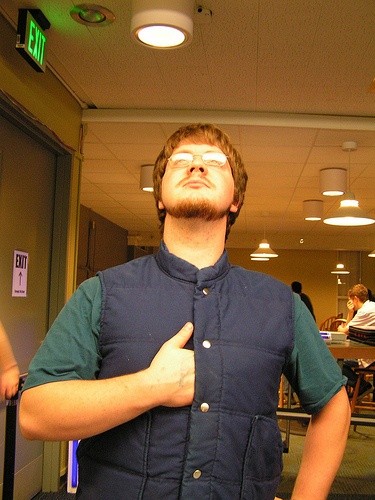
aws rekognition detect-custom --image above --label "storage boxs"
[332,332,347,343]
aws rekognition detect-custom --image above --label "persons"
[291,281,317,323]
[19,122,352,500]
[0,323,20,402]
[337,284,375,400]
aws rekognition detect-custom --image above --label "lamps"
[140,165,156,193]
[322,140,375,227]
[128,0,196,50]
[250,210,279,262]
[303,200,324,222]
[367,248,375,258]
[331,249,352,275]
[320,168,348,197]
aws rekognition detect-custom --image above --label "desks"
[324,339,375,360]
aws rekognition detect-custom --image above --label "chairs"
[319,316,336,331]
[330,318,347,332]
[337,312,344,319]
[350,361,375,432]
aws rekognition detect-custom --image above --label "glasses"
[168,152,230,168]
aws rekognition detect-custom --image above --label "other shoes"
[348,382,374,401]
[283,400,297,405]
